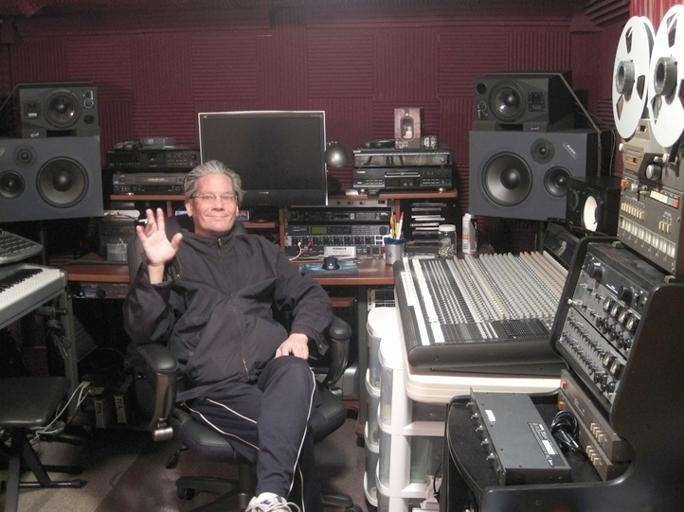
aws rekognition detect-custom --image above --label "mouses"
[321,254,339,271]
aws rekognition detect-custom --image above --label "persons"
[122,159,334,512]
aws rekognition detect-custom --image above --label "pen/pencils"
[387,211,404,240]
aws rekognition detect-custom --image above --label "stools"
[2,377,85,511]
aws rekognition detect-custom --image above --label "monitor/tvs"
[196,110,328,209]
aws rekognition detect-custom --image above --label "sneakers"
[244,491,295,512]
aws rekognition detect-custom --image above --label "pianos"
[0,262,68,333]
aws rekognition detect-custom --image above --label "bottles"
[438,225,457,260]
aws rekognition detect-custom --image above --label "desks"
[60,183,460,439]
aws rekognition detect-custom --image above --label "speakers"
[0,79,103,136]
[473,68,572,133]
[0,135,105,223]
[468,130,614,221]
[563,176,619,236]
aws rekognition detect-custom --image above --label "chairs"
[124,217,364,512]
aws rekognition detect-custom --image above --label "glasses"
[188,193,239,202]
